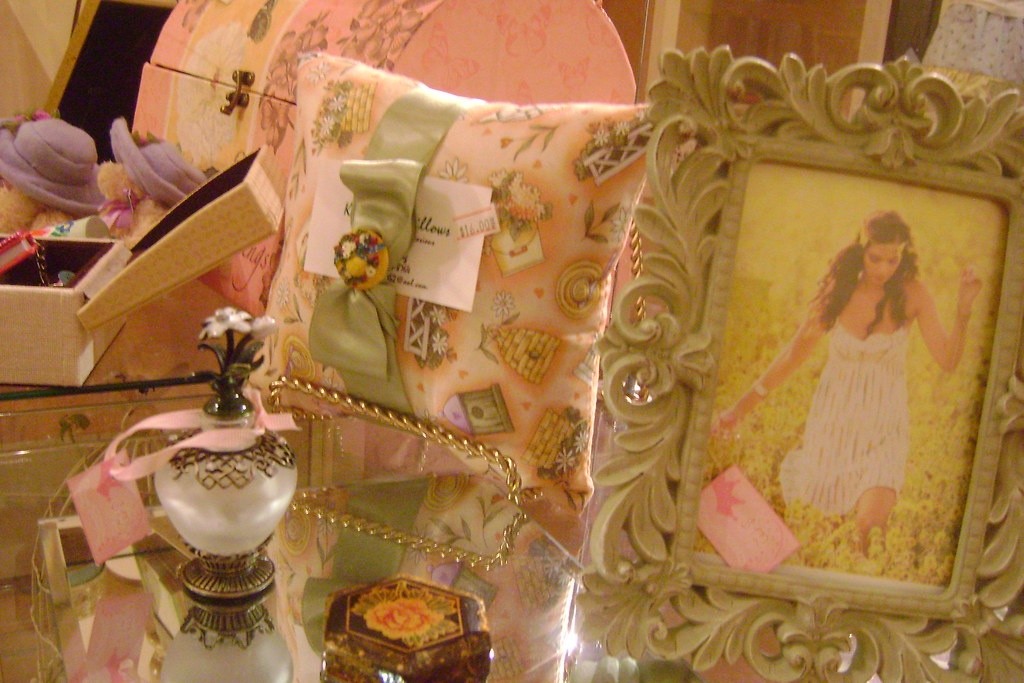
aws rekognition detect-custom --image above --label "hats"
[2,117,107,215]
[111,117,206,204]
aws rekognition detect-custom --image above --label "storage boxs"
[2,148,283,391]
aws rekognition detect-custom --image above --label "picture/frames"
[571,49,1024,682]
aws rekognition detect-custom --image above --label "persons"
[712,213,984,557]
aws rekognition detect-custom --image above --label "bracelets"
[753,382,769,399]
